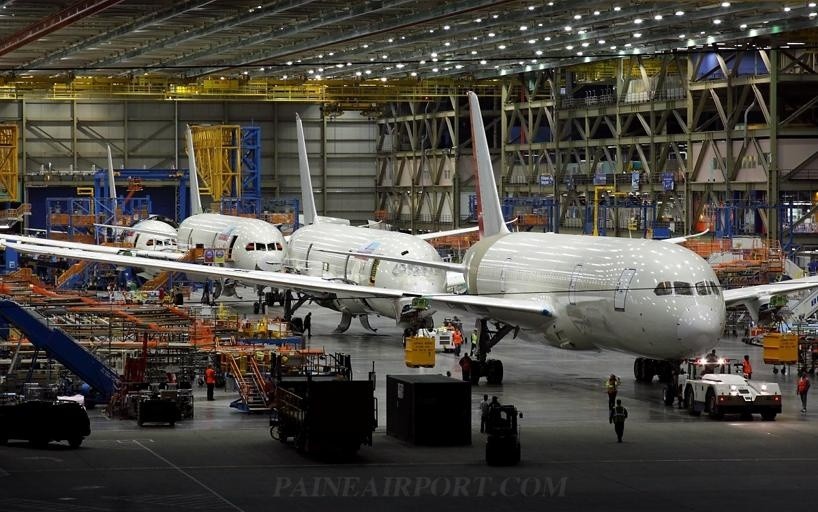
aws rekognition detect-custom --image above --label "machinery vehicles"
[658,356,786,422]
[482,404,523,466]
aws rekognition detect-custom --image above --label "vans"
[1,398,91,448]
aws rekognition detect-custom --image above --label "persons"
[117,374,128,420]
[660,356,678,408]
[451,327,463,359]
[479,394,490,433]
[704,349,720,372]
[796,371,810,414]
[458,352,472,381]
[742,354,753,378]
[173,287,183,305]
[488,396,501,418]
[605,372,622,410]
[609,399,628,443]
[158,286,168,302]
[402,327,413,348]
[468,327,477,359]
[446,321,455,331]
[301,312,312,338]
[447,370,451,377]
[205,363,216,400]
